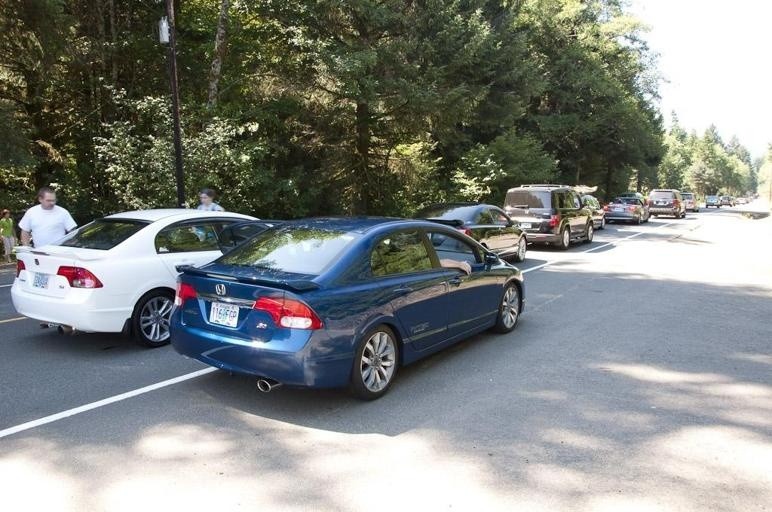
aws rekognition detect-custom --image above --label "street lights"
[156,0,185,208]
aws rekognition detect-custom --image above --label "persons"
[0,209,15,263]
[189,188,225,242]
[18,187,79,327]
[440,258,471,274]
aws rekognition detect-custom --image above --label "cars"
[705,191,761,209]
[503,181,701,251]
